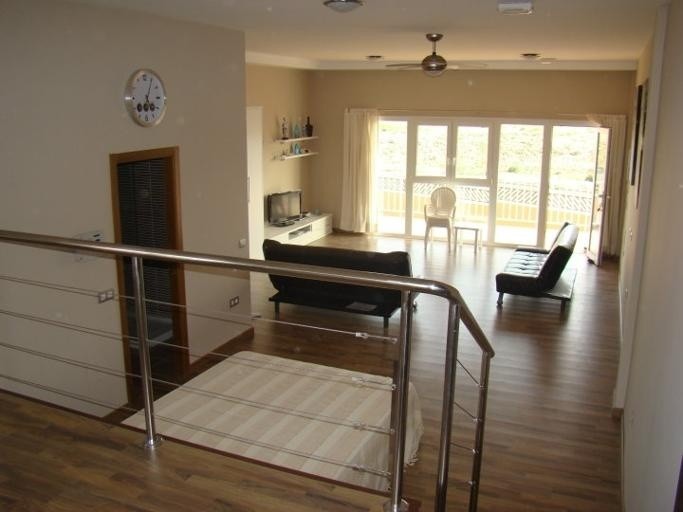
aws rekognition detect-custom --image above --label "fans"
[387,34,489,71]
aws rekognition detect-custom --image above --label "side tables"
[454,226,482,253]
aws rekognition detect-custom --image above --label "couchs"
[494,221,580,316]
[262,237,424,328]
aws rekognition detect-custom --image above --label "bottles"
[305,116,313,137]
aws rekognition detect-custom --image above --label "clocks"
[123,68,168,127]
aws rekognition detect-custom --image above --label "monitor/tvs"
[267,191,303,227]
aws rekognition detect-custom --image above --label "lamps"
[422,53,446,76]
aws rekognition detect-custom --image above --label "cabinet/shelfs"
[273,135,319,161]
[265,213,332,246]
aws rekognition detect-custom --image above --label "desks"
[118,349,423,494]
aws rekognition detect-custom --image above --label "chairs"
[423,185,456,253]
